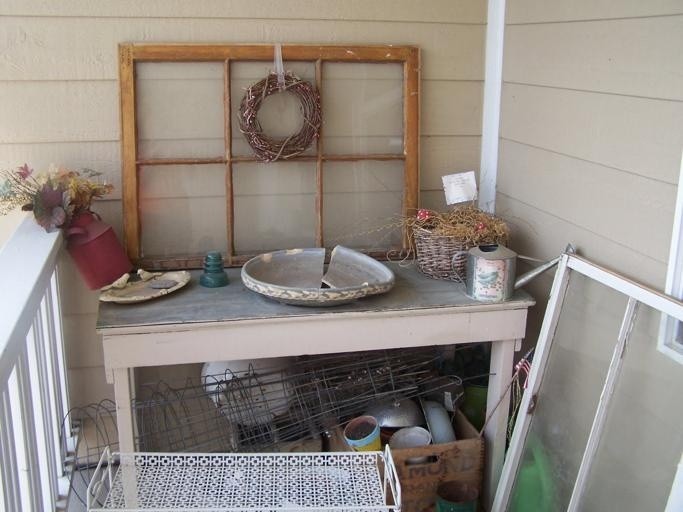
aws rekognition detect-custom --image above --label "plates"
[96,269,192,306]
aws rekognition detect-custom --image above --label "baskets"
[413,213,505,281]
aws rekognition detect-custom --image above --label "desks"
[93,256,537,512]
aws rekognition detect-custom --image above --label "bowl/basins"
[418,394,458,444]
[236,243,400,308]
[200,357,307,427]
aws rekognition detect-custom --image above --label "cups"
[386,425,432,467]
[342,414,381,449]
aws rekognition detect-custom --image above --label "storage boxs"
[321,385,487,512]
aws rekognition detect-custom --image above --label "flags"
[515,346,533,388]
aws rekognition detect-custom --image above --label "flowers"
[0,161,114,233]
